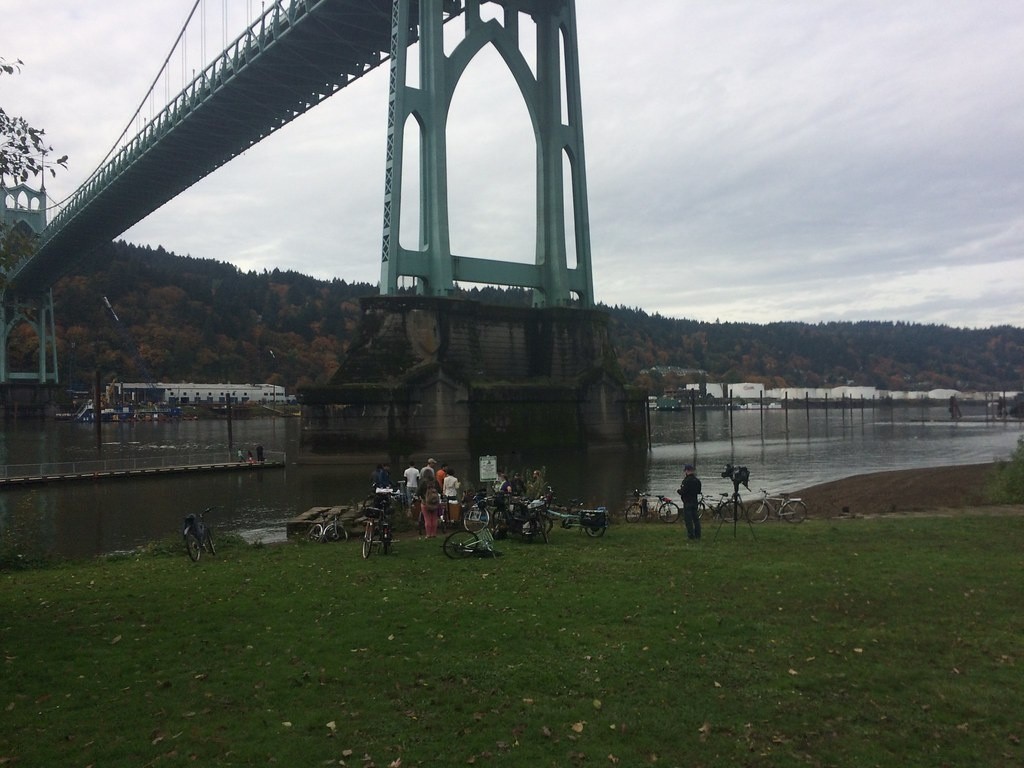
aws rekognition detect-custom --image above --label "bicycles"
[418,493,455,534]
[535,486,609,537]
[747,488,808,524]
[362,499,393,559]
[183,508,216,562]
[307,513,348,545]
[626,488,680,523]
[683,492,743,523]
[464,486,554,543]
[441,523,501,560]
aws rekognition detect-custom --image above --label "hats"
[683,465,693,471]
[428,458,437,464]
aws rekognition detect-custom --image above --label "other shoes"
[684,537,692,541]
[432,536,436,538]
[693,537,700,541]
[425,535,431,539]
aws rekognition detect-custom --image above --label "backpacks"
[424,477,439,511]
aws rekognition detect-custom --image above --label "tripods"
[713,482,757,543]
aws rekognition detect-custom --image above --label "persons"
[255,443,264,461]
[368,458,545,539]
[246,450,254,463]
[237,449,242,462]
[677,465,702,539]
